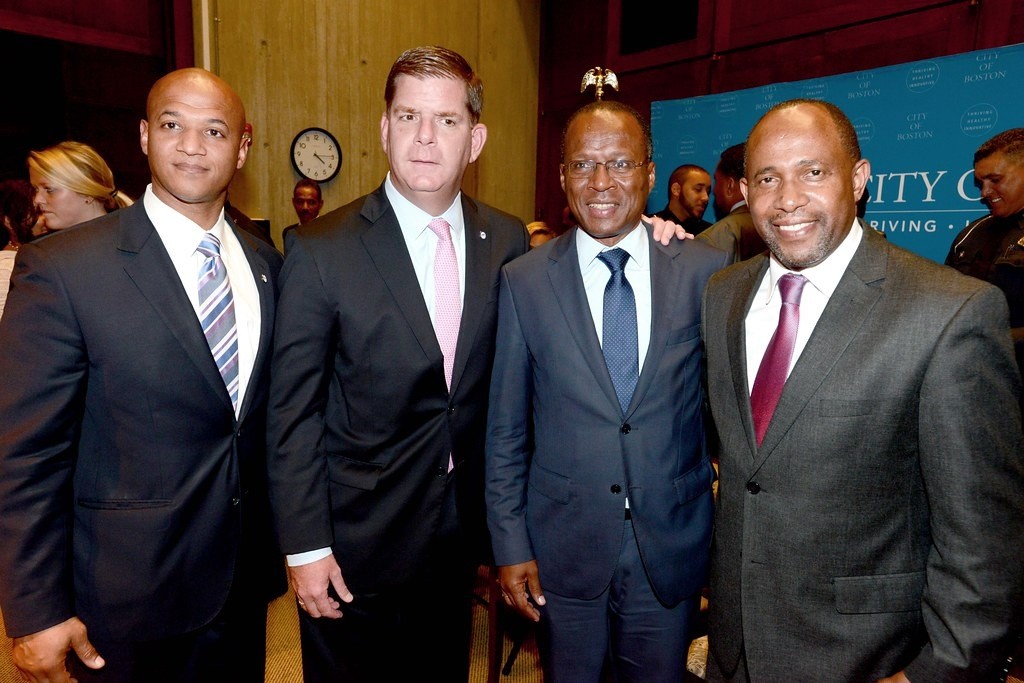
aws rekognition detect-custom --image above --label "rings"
[299,600,305,607]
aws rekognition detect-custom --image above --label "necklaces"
[8,241,19,251]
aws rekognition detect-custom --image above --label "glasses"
[561,157,649,180]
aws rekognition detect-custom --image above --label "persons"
[697,141,768,265]
[526,222,558,251]
[273,46,693,683]
[0,139,136,324]
[282,179,325,242]
[698,99,1023,683]
[486,101,732,683]
[648,164,714,238]
[943,128,1024,378]
[0,65,285,683]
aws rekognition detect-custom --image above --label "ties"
[196,234,240,414]
[750,273,810,449]
[427,218,462,394]
[595,248,640,418]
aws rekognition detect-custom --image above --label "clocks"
[290,126,343,183]
